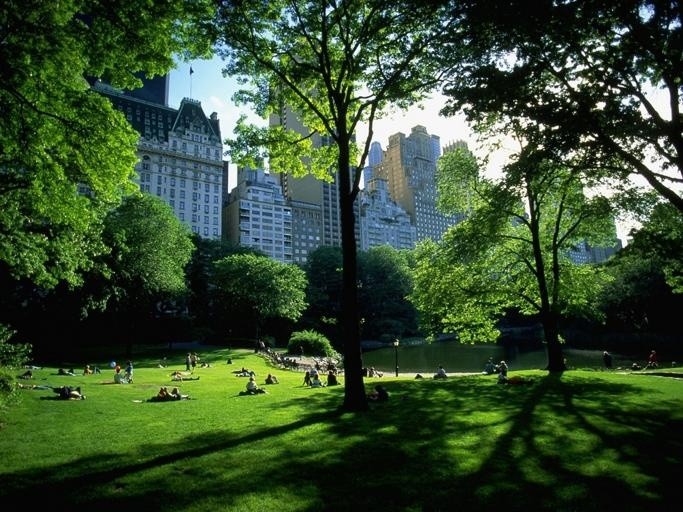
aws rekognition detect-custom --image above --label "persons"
[17,358,134,401]
[370,384,388,403]
[146,351,213,401]
[368,367,374,377]
[484,358,509,384]
[434,365,446,378]
[233,338,344,396]
[631,350,677,370]
[603,351,613,368]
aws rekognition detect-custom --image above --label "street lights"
[392,338,400,378]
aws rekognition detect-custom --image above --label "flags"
[190,67,193,75]
